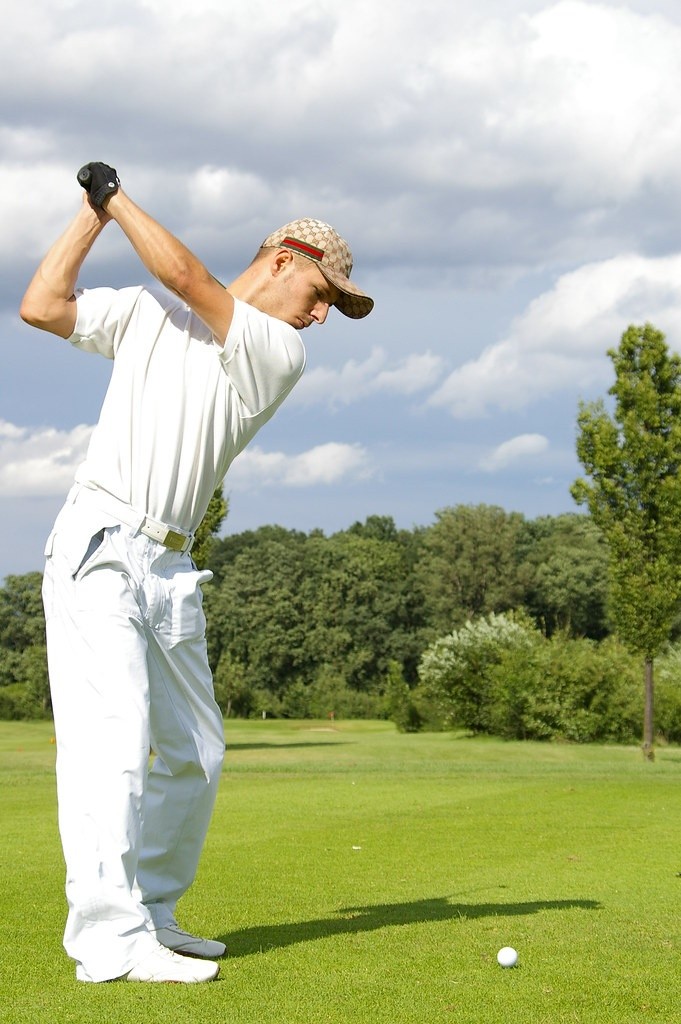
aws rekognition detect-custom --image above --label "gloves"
[76,161,120,208]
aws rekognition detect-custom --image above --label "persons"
[19,163,375,984]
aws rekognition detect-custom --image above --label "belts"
[66,484,193,553]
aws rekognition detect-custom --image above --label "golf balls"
[497,946,519,968]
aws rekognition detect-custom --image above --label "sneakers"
[117,937,220,984]
[147,919,227,958]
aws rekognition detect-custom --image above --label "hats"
[260,218,375,321]
